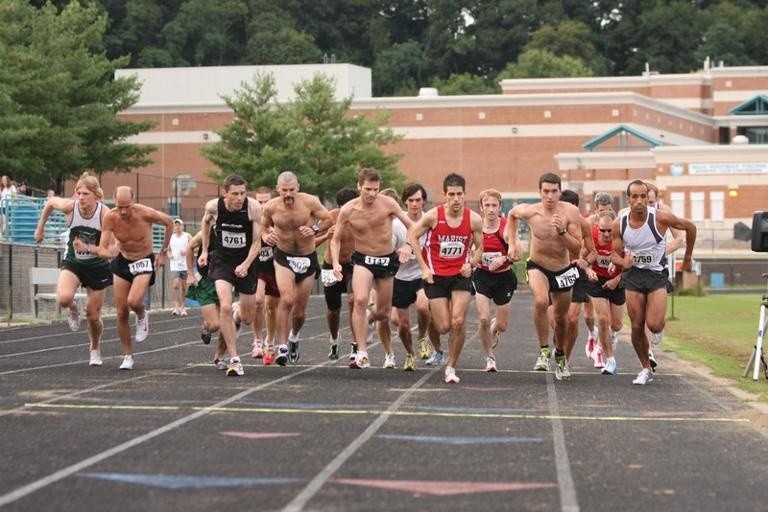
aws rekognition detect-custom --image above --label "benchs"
[28,266,88,318]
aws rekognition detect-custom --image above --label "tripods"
[743,294,768,381]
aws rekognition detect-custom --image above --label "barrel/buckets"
[711,270,725,288]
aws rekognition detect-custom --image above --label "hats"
[173,218,184,226]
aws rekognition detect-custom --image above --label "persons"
[507,167,698,386]
[0,167,198,371]
[184,167,521,386]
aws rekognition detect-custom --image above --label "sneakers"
[484,318,501,372]
[89,343,103,367]
[202,301,300,374]
[171,308,189,317]
[585,324,620,376]
[119,356,135,370]
[382,339,463,384]
[135,310,150,344]
[326,307,375,368]
[533,348,551,373]
[552,348,571,381]
[65,301,81,332]
[632,330,663,386]
